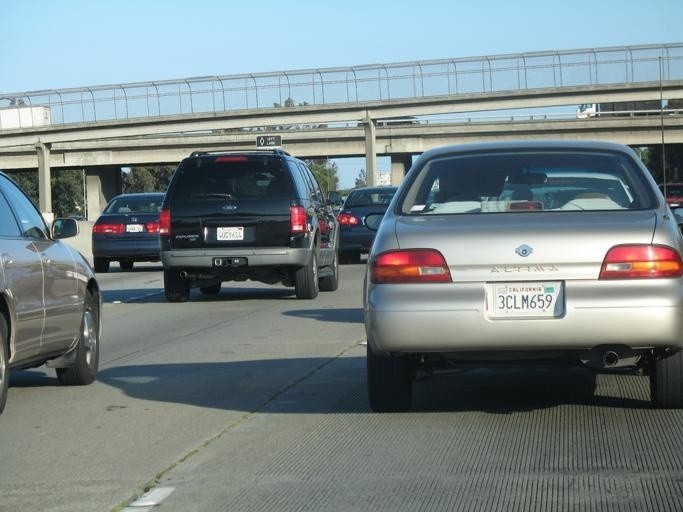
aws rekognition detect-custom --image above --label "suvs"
[158,145,343,304]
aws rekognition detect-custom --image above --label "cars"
[359,136,682,413]
[91,192,166,274]
[0,169,104,416]
[334,183,399,265]
[333,141,682,215]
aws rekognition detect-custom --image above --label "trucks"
[577,98,682,119]
